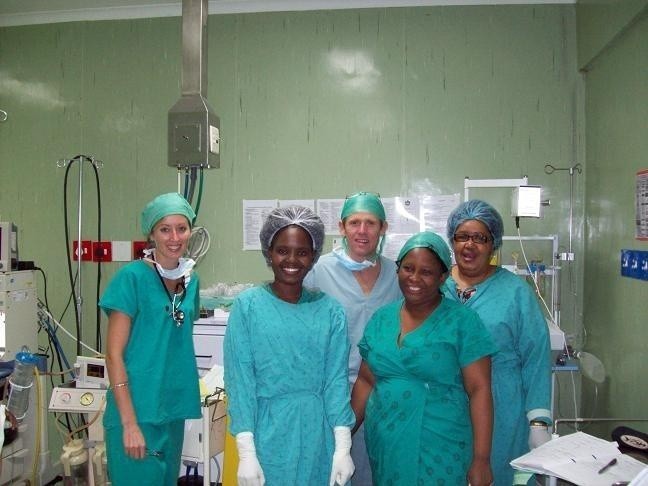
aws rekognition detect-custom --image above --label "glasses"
[345,192,381,204]
[454,232,493,243]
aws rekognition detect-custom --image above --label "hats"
[141,192,196,236]
[398,232,452,272]
[341,193,385,221]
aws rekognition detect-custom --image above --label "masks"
[143,248,196,280]
[333,246,376,271]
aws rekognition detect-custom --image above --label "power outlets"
[134,240,150,260]
[72,240,93,261]
[92,242,111,262]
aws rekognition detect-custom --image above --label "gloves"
[236,432,266,486]
[528,428,551,451]
[329,426,355,486]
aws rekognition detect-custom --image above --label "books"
[508,428,648,485]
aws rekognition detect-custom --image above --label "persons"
[97,192,207,486]
[441,198,556,486]
[304,189,405,486]
[0,403,18,458]
[221,202,356,486]
[349,229,496,486]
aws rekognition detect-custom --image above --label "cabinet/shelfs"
[181,313,227,483]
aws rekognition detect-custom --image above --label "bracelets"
[528,421,552,430]
[107,380,131,389]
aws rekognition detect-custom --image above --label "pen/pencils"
[598,459,616,474]
